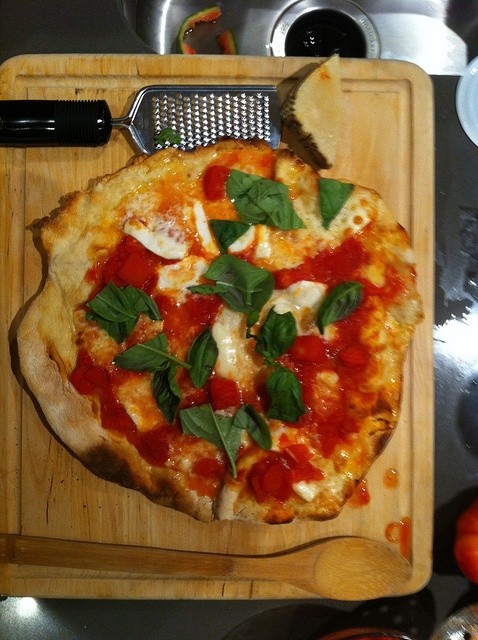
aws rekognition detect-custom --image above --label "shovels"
[0,85,280,156]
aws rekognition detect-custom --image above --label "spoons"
[0,531,415,603]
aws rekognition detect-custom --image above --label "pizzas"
[17,136,425,526]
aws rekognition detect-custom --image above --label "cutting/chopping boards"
[0,51,437,602]
